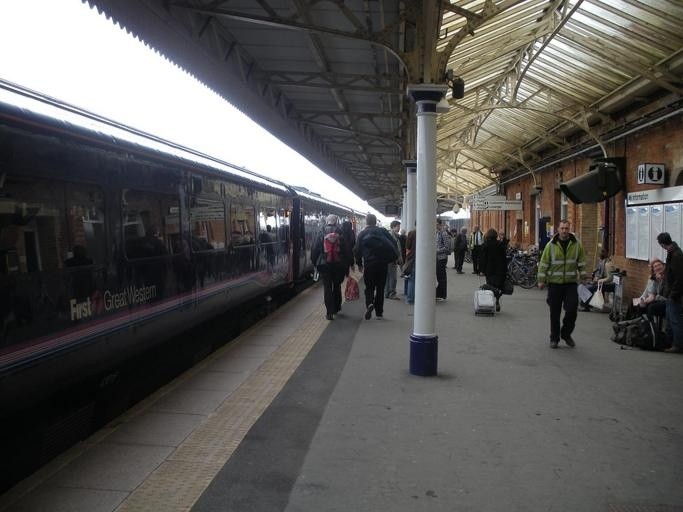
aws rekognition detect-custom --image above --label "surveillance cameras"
[452,77,464,99]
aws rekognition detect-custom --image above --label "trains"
[0,78,382,498]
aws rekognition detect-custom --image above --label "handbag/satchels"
[589,290,604,310]
[642,322,667,352]
[612,318,645,347]
[345,277,359,301]
[502,278,514,295]
[363,229,399,264]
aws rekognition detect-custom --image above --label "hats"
[326,215,338,225]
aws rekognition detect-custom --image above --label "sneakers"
[365,304,374,320]
[563,335,575,347]
[550,341,557,348]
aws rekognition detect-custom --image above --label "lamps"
[444,67,465,100]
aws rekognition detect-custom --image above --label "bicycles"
[465,241,544,289]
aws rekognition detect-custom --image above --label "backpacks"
[322,226,342,264]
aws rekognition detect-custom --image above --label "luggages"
[473,278,496,317]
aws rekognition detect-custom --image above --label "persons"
[626,258,666,327]
[656,232,682,354]
[577,245,613,312]
[193,218,288,283]
[537,221,586,349]
[312,211,513,320]
